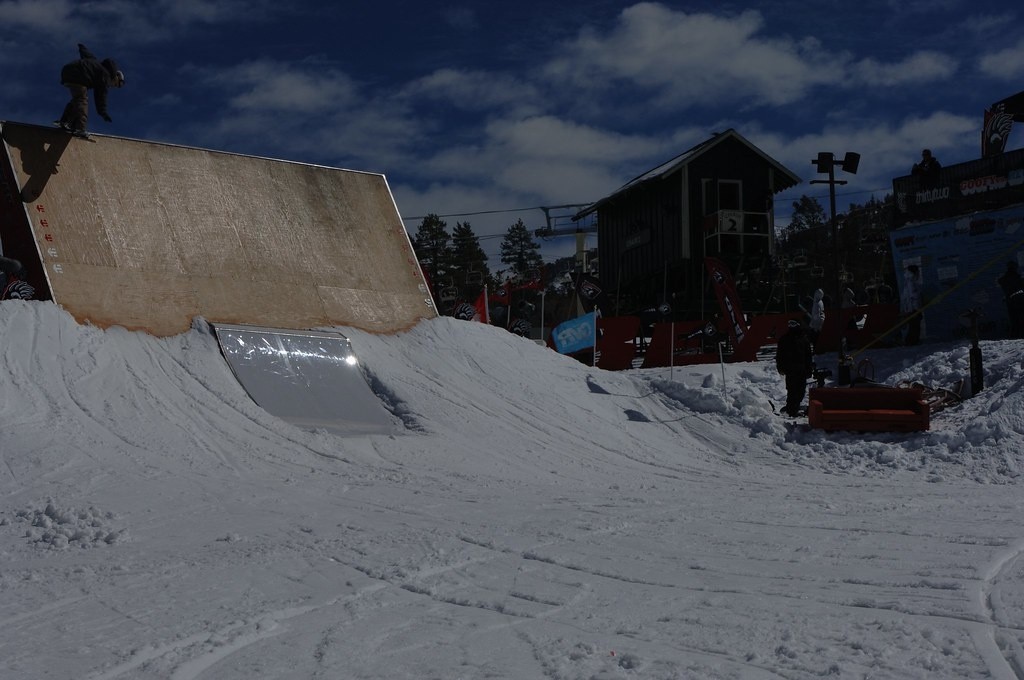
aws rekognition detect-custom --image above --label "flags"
[474,264,601,355]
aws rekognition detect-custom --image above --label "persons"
[52,43,125,138]
[900,265,923,346]
[776,288,825,416]
[998,261,1024,340]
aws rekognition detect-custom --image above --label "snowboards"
[53,121,96,143]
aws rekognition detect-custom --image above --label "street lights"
[809,152,860,353]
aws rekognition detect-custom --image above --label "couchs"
[808,387,930,431]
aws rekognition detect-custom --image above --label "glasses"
[788,320,798,328]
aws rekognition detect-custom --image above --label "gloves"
[778,366,784,374]
[808,368,816,378]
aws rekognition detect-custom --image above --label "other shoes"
[789,411,802,417]
